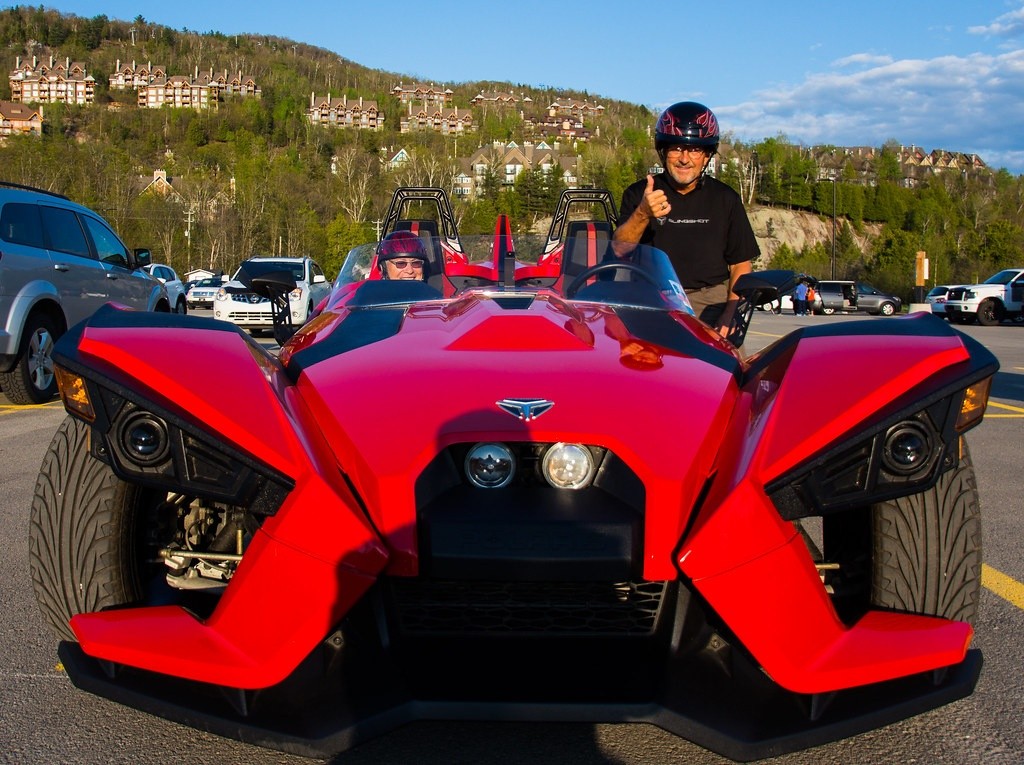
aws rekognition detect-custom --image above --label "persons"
[790,278,814,317]
[376,230,427,281]
[610,101,761,338]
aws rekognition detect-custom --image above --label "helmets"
[655,102,719,152]
[377,231,430,271]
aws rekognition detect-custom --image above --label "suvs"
[213,253,331,336]
[0,180,174,404]
[945,269,1024,324]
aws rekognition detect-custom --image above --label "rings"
[661,202,666,211]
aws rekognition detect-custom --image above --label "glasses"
[389,259,423,269]
[666,148,705,159]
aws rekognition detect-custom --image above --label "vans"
[810,279,902,315]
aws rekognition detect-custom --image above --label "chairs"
[534,189,620,301]
[372,187,468,299]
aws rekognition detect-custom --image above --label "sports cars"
[29,180,1003,723]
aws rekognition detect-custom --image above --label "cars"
[756,290,793,311]
[143,265,187,315]
[926,284,966,317]
[187,279,224,310]
[184,282,199,293]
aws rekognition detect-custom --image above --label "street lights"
[820,178,836,280]
